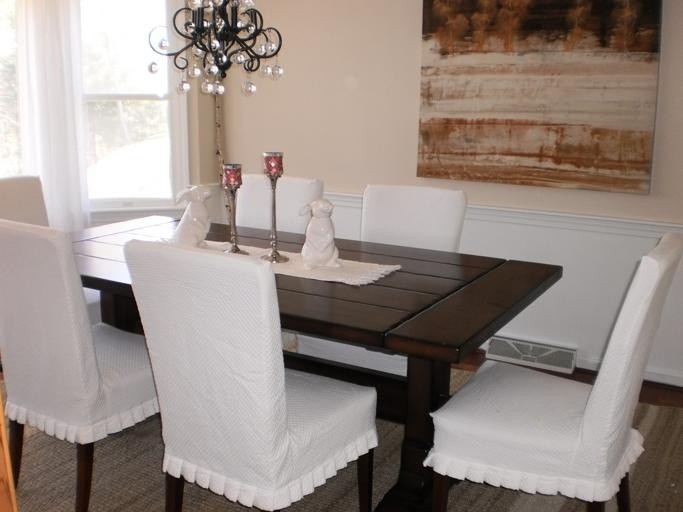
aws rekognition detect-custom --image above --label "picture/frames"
[415,0,664,194]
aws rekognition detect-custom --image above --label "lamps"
[146,0,286,98]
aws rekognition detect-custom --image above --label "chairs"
[0,175,49,227]
[236,173,323,235]
[422,226,683,512]
[2,219,159,511]
[123,240,378,511]
[295,184,471,376]
